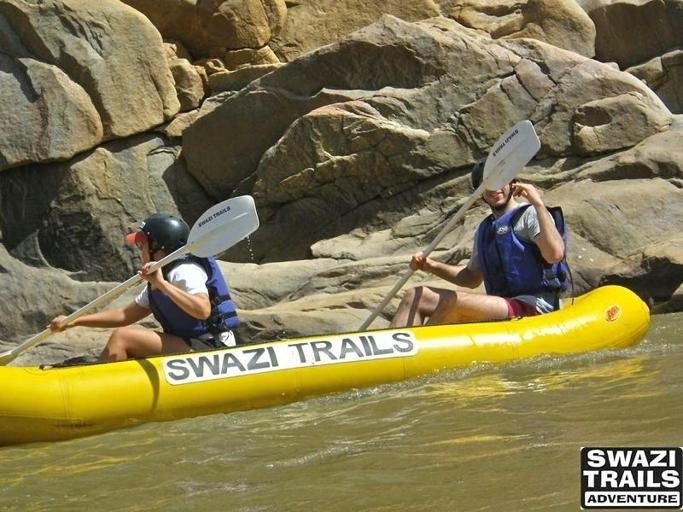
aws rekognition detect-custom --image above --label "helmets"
[125,212,191,254]
[471,157,488,190]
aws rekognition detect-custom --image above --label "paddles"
[359,120,542,332]
[1,194,260,365]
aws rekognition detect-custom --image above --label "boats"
[0,284,651,447]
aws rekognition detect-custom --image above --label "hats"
[126,231,147,246]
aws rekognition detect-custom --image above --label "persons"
[50,213,240,363]
[389,156,569,328]
